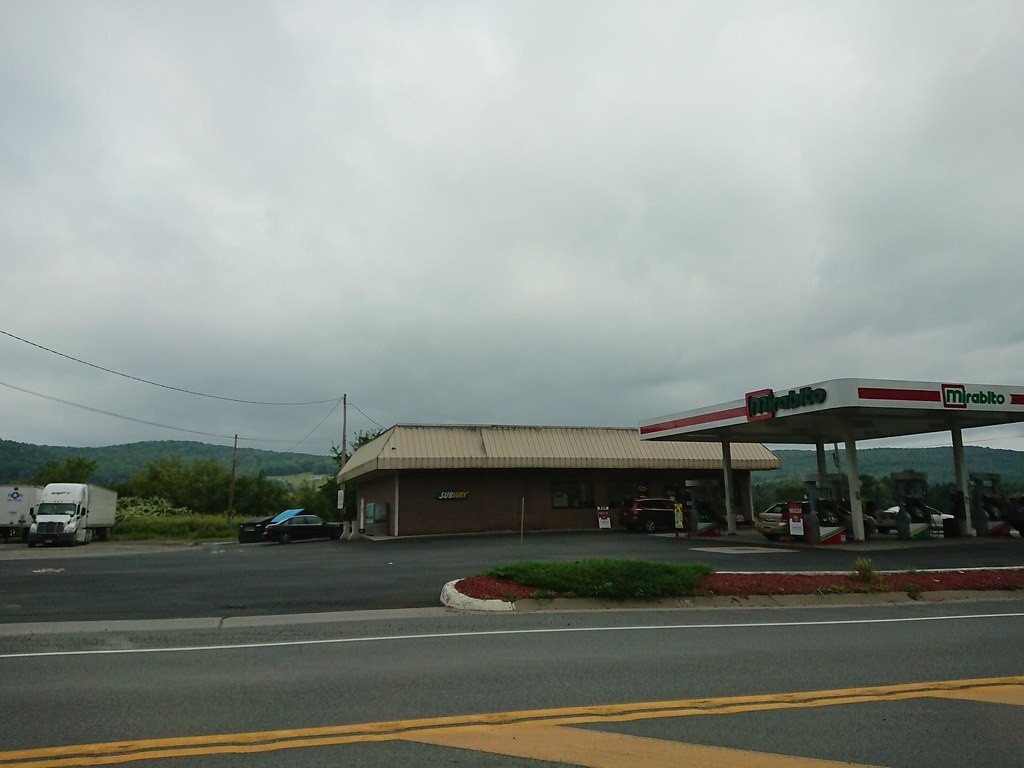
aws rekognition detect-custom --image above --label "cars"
[621,498,692,535]
[264,515,344,544]
[876,504,955,534]
[755,499,877,541]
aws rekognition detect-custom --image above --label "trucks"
[0,484,45,542]
[28,483,118,548]
